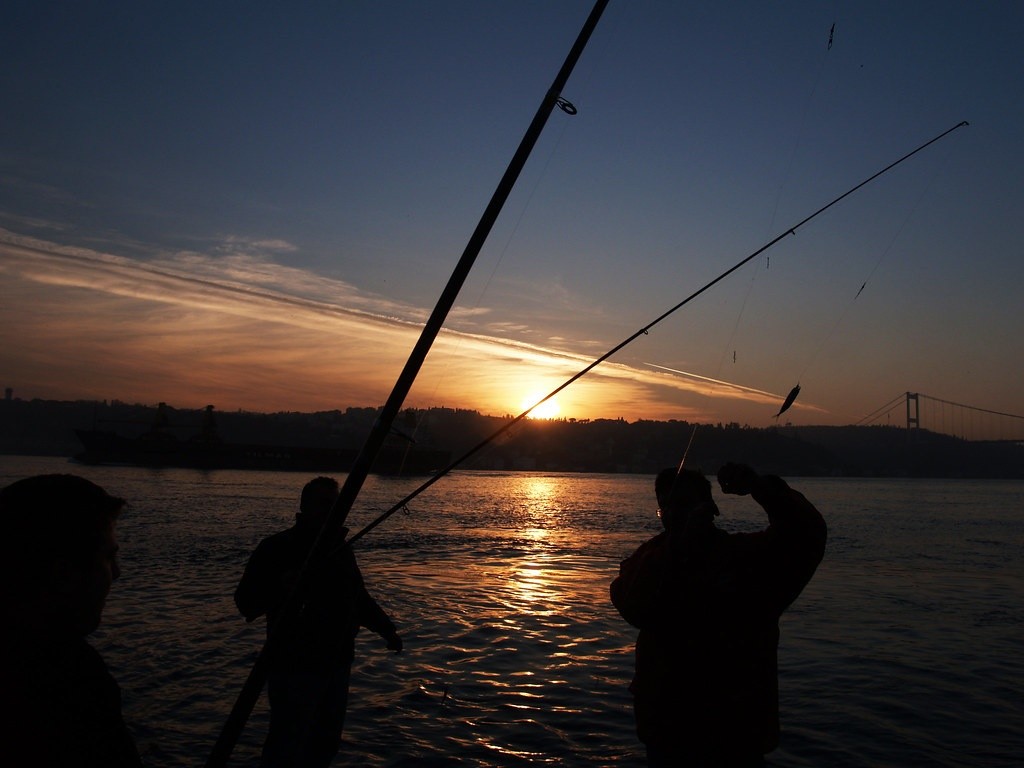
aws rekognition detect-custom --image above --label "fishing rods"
[346,120,971,552]
[206,1,611,767]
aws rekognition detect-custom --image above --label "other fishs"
[771,384,802,423]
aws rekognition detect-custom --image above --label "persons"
[606,456,829,768]
[0,468,148,768]
[232,474,407,768]
